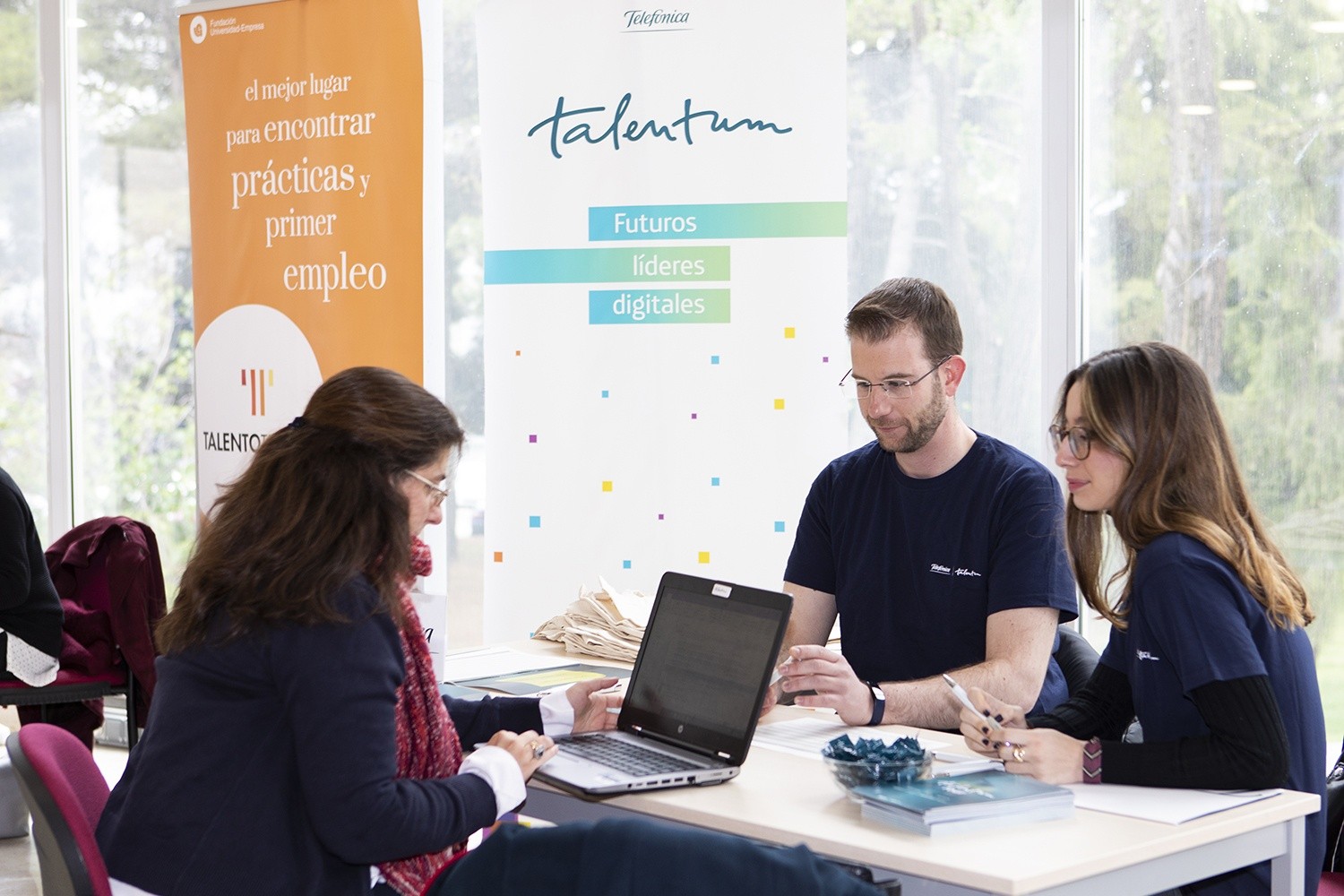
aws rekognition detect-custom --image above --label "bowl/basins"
[822,752,936,805]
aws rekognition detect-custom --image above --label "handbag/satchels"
[1322,736,1344,872]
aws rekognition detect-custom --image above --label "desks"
[431,641,1322,896]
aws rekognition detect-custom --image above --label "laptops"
[474,572,795,800]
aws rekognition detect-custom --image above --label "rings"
[1013,746,1024,762]
[529,739,545,758]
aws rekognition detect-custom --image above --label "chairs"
[0,516,1344,896]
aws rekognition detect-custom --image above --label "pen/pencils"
[943,674,1009,746]
[769,656,794,686]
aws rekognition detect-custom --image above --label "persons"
[957,343,1330,896]
[715,272,1084,730]
[95,363,632,896]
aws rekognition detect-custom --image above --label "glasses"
[840,354,953,399]
[400,468,449,508]
[1050,424,1103,460]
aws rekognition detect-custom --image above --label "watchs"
[861,678,886,726]
